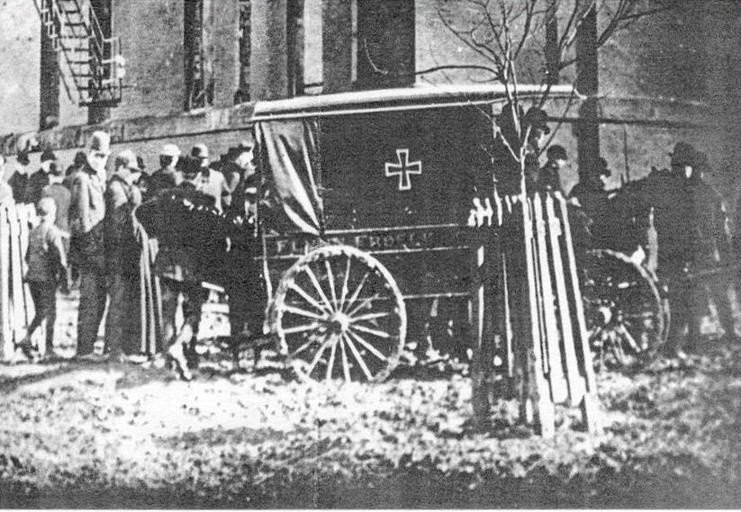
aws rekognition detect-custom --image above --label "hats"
[599,156,611,176]
[548,145,567,161]
[115,150,141,171]
[192,144,209,158]
[160,143,181,156]
[87,131,110,154]
[495,104,550,134]
[669,143,712,172]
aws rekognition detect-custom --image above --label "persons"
[1,129,260,380]
[502,110,739,359]
